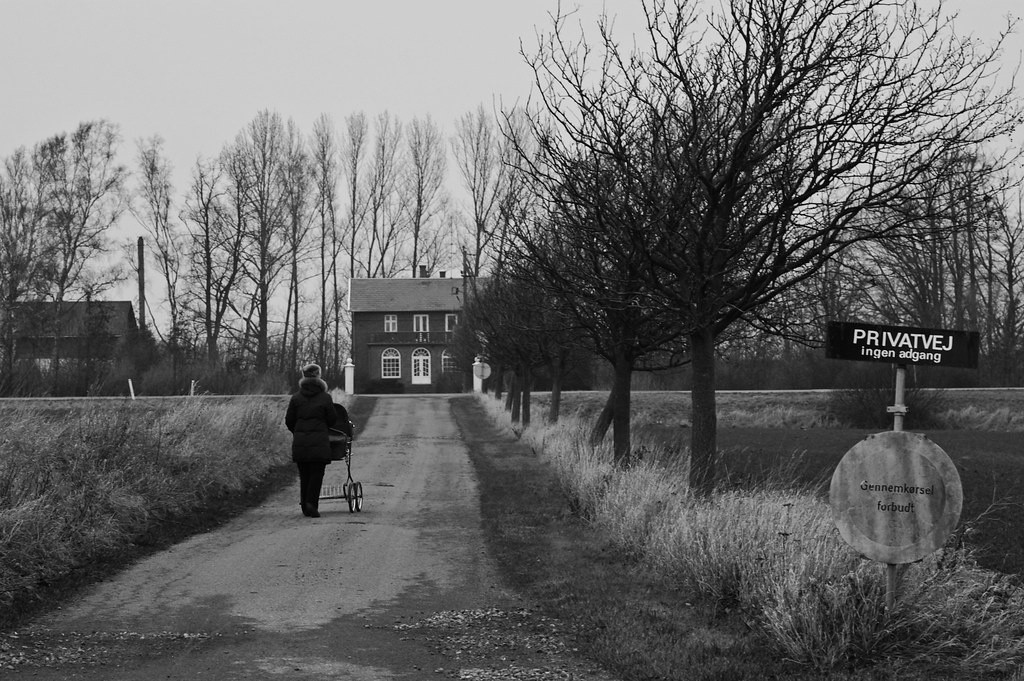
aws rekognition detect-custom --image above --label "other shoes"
[303,504,320,518]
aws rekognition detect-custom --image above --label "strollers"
[299,402,363,516]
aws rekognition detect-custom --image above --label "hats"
[302,364,321,379]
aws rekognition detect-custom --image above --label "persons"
[285,364,337,517]
